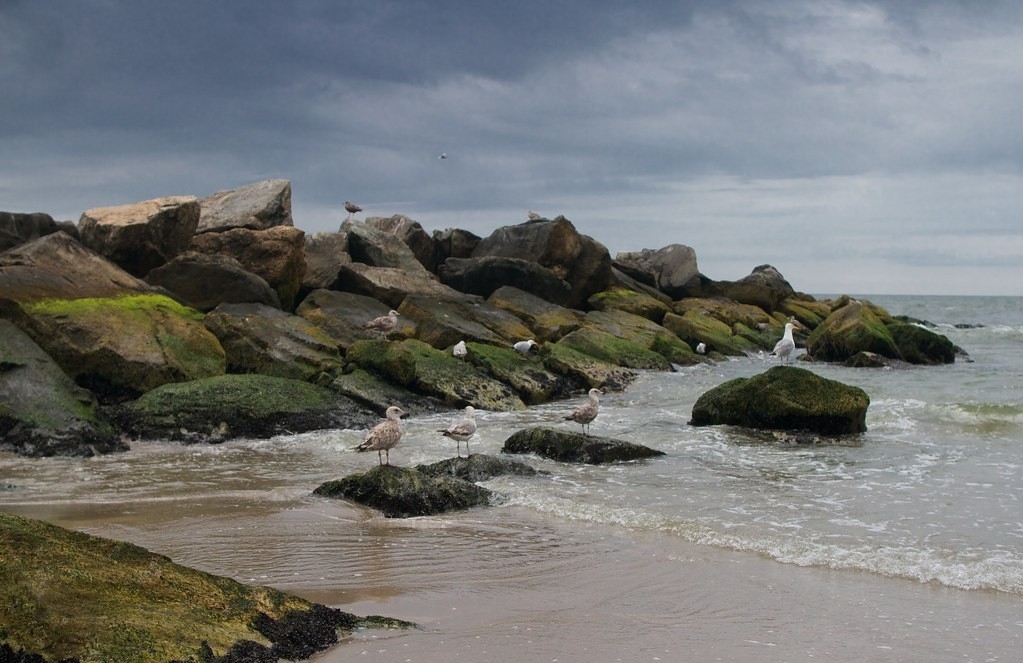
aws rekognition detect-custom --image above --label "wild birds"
[436,406,477,458]
[342,200,363,218]
[769,323,799,366]
[453,341,467,361]
[696,343,706,354]
[513,340,537,357]
[349,406,406,466]
[528,210,540,219]
[362,310,400,342]
[438,153,448,159]
[561,388,603,435]
